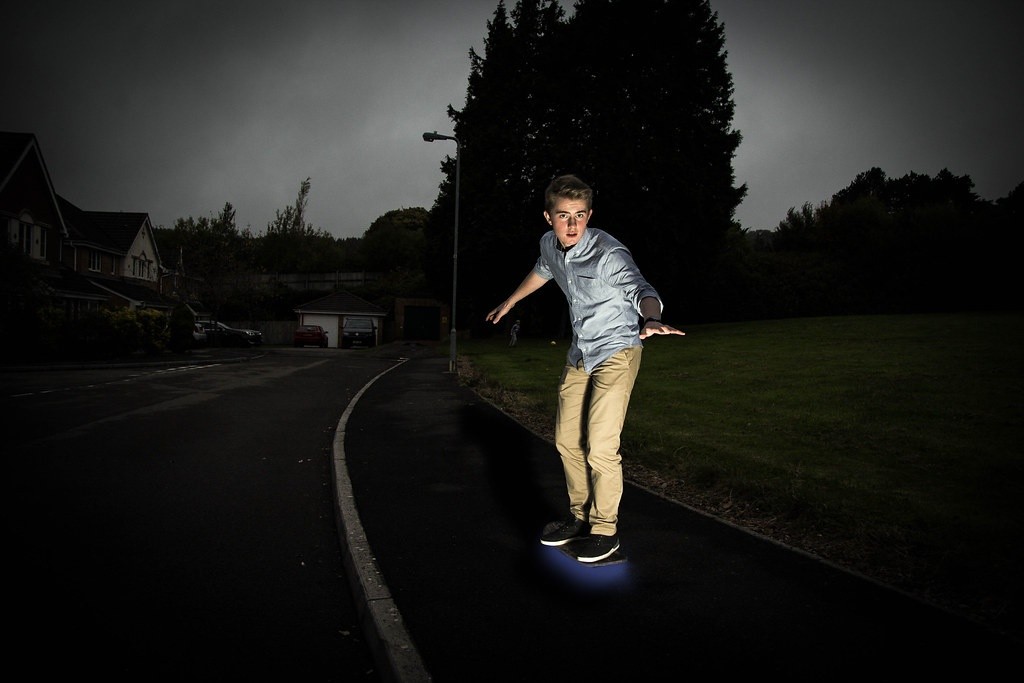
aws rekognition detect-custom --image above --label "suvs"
[340,316,378,348]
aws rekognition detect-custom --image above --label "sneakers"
[577,532,620,563]
[540,509,590,546]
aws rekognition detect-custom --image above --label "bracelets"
[643,317,664,329]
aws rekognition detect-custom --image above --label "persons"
[486,176,685,565]
[508,319,520,347]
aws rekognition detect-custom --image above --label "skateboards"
[552,525,629,570]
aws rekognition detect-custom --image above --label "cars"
[296,324,329,348]
[198,320,264,349]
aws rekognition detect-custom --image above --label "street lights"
[423,131,461,373]
